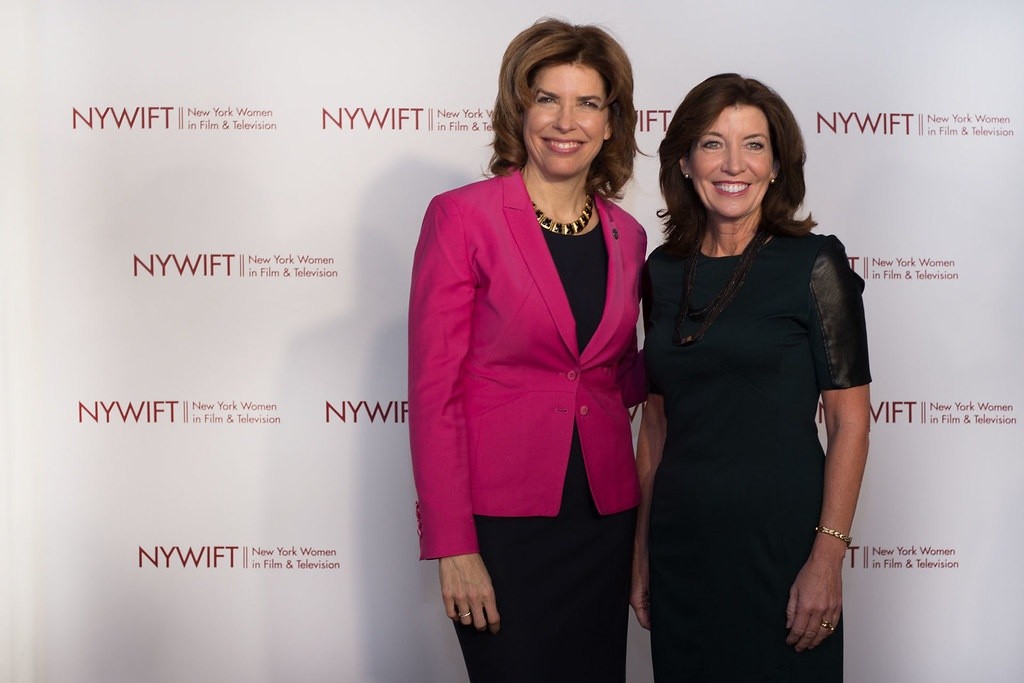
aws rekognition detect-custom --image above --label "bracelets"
[815,526,852,547]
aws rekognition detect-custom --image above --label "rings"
[458,611,471,618]
[820,619,834,631]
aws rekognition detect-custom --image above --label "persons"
[629,74,873,683]
[408,16,649,683]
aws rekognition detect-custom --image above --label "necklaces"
[531,184,595,234]
[673,218,775,345]
